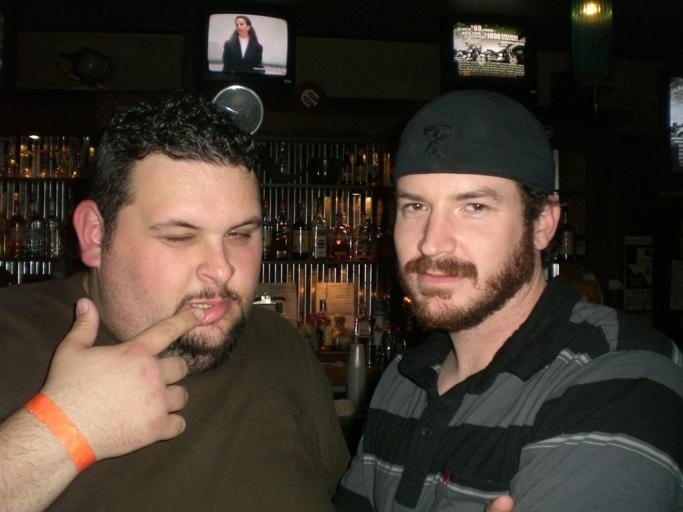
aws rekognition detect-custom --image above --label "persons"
[0,92,357,512]
[222,14,264,74]
[321,86,681,511]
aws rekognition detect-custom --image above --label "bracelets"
[24,389,97,472]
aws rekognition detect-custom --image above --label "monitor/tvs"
[182,0,297,104]
[442,10,540,99]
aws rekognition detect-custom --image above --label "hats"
[390,88,559,192]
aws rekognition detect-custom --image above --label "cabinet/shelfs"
[1,134,431,366]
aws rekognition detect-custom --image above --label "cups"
[353,141,382,187]
[0,132,94,179]
[345,343,368,410]
[307,151,345,184]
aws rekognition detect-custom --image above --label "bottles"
[555,224,580,272]
[317,294,410,369]
[271,139,291,184]
[260,197,387,260]
[5,190,62,258]
